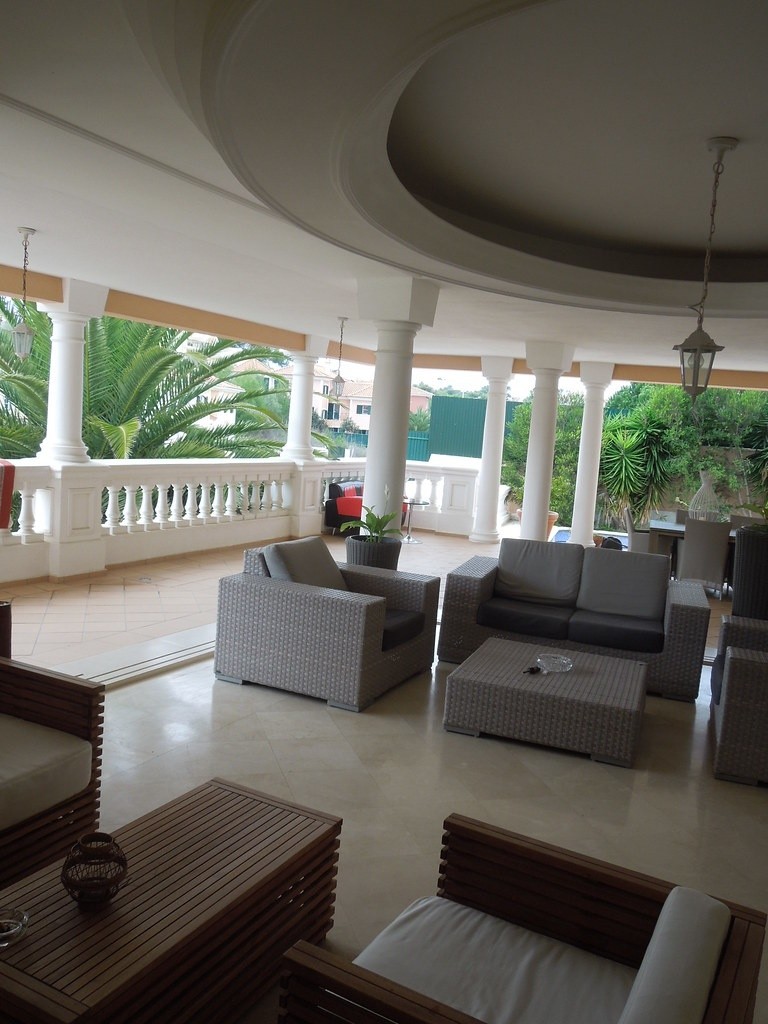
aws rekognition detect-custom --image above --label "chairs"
[0,655,106,891]
[275,813,768,1024]
[623,507,650,553]
[676,509,719,524]
[729,514,767,531]
[675,520,732,602]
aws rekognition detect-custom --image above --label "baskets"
[60,832,132,907]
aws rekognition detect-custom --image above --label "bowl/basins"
[0,908,30,950]
[536,654,573,674]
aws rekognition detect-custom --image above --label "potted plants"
[339,504,404,571]
[512,484,559,537]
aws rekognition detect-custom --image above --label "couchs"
[436,538,711,703]
[710,614,768,789]
[323,479,409,535]
[213,536,441,713]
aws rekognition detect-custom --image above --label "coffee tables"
[441,636,649,769]
[0,776,343,1024]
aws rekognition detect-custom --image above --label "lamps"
[331,316,349,400]
[672,135,738,405]
[12,225,36,364]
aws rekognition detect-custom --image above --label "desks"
[401,498,429,544]
[648,519,736,557]
